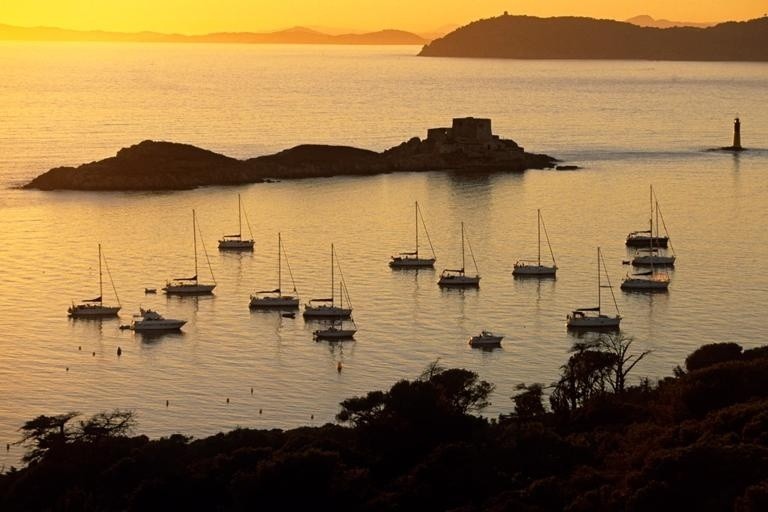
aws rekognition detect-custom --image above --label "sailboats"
[313,283,358,338]
[302,241,356,318]
[66,242,122,315]
[218,193,255,248]
[470,330,504,346]
[632,202,677,266]
[436,221,481,285]
[160,208,218,292]
[248,231,300,307]
[388,200,438,266]
[508,209,559,276]
[128,305,191,331]
[619,218,673,290]
[565,245,623,327]
[621,181,670,247]
